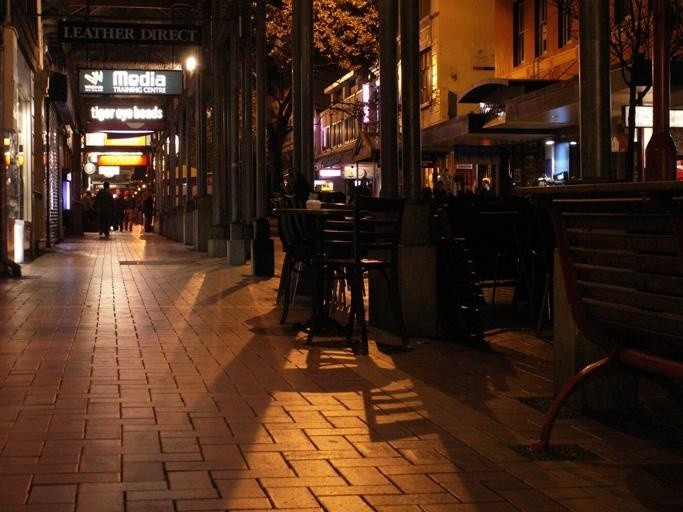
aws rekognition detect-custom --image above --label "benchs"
[537,193,681,455]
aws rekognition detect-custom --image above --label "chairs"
[270,191,407,357]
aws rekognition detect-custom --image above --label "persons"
[79,181,155,236]
[479,180,496,198]
[433,180,448,198]
